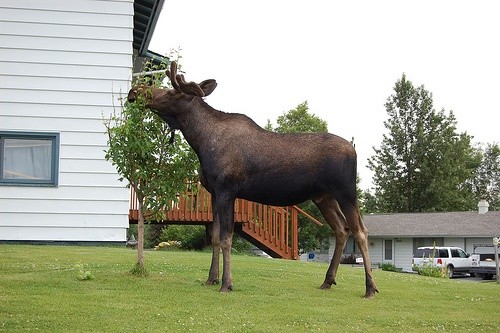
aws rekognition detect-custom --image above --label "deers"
[127,61,379,299]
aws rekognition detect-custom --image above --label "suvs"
[411,246,475,279]
